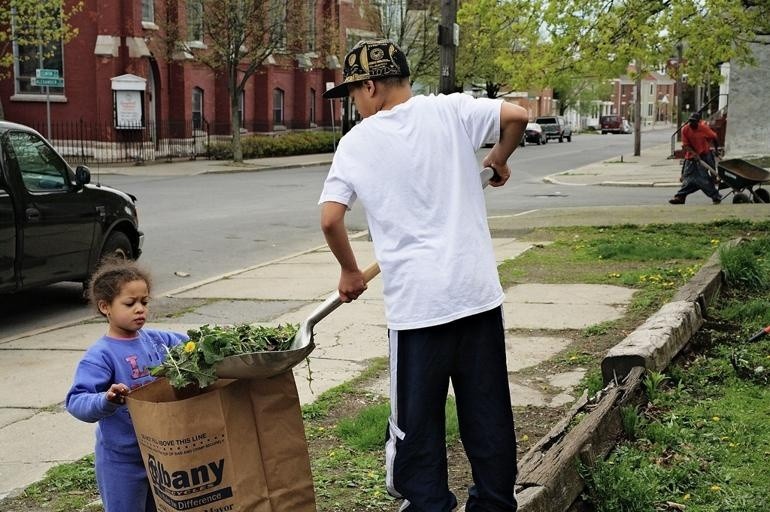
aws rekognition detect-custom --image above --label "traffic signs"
[30,77,66,88]
[36,69,61,80]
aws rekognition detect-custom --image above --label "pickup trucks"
[0,120,146,303]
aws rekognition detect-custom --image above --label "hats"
[689,113,700,120]
[320,38,410,98]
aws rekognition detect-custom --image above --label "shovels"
[216,167,496,379]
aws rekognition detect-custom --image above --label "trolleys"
[697,149,770,205]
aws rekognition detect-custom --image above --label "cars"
[623,119,633,134]
[484,114,573,148]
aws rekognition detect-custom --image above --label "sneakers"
[713,195,721,204]
[669,195,685,203]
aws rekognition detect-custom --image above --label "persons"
[63,248,197,512]
[314,35,532,512]
[667,110,723,206]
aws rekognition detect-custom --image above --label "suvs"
[600,115,623,134]
